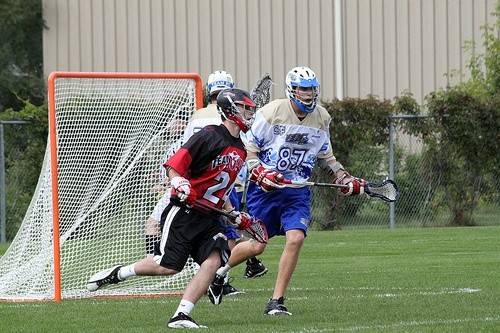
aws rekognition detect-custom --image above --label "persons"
[144,69,269,296]
[86,88,257,327]
[209,66,368,317]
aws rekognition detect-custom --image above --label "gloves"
[332,171,367,197]
[226,209,255,230]
[250,165,286,193]
[171,176,197,208]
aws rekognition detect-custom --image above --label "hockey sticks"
[249,73,272,111]
[171,187,269,246]
[278,179,398,202]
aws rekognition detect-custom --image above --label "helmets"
[205,70,234,98]
[284,67,320,113]
[217,88,257,134]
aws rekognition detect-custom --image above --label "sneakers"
[207,273,226,306]
[263,296,292,316]
[222,281,247,297]
[242,260,268,279]
[86,265,125,292]
[166,311,208,329]
